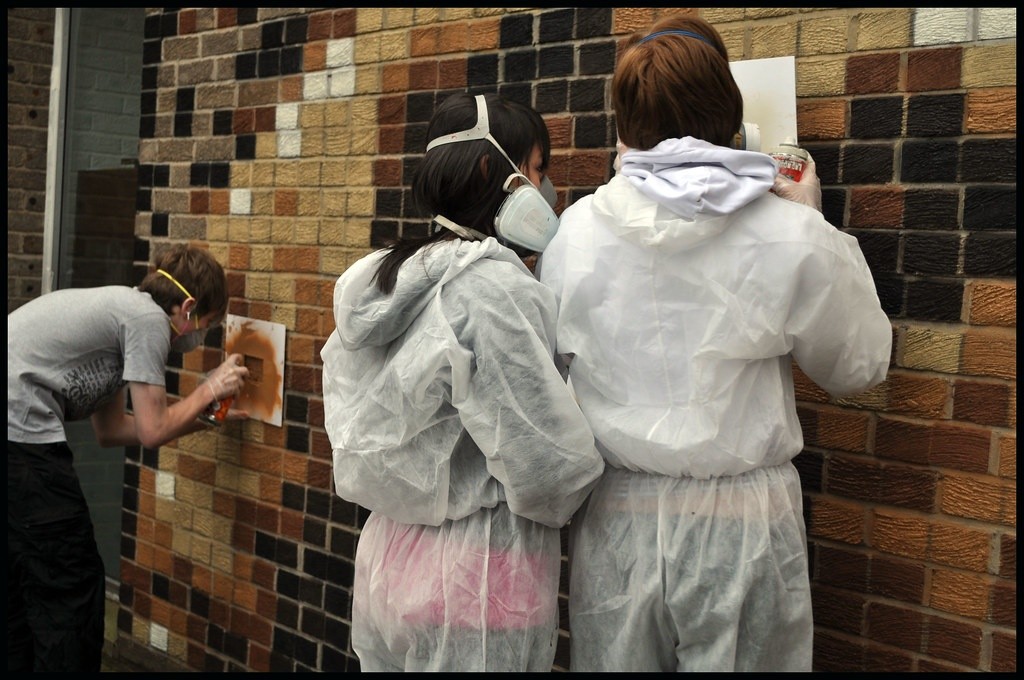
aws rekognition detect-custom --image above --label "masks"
[173,327,209,353]
[539,174,558,209]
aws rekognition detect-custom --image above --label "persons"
[534,14,891,671]
[320,84,606,672]
[8,243,249,680]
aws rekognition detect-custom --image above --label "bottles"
[767,137,808,196]
[198,361,243,427]
